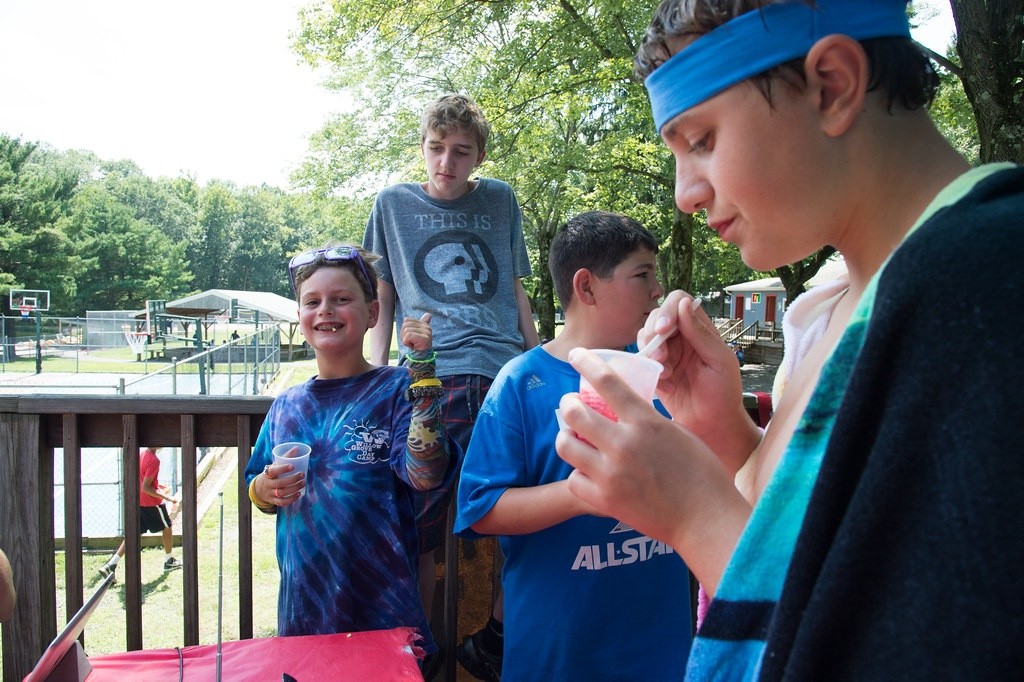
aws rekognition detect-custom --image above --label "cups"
[578,348,663,445]
[272,442,312,497]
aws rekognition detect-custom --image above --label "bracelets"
[403,377,445,400]
[404,350,439,364]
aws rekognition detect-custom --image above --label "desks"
[86,627,426,681]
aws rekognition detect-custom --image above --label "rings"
[262,464,275,479]
[273,487,283,500]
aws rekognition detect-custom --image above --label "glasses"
[288,245,375,301]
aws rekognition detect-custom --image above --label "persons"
[362,97,545,659]
[97,447,182,587]
[241,235,450,680]
[445,210,692,682]
[551,0,1024,681]
[191,328,259,358]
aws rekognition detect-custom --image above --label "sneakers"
[457,629,504,681]
[98,564,118,584]
[164,557,183,572]
